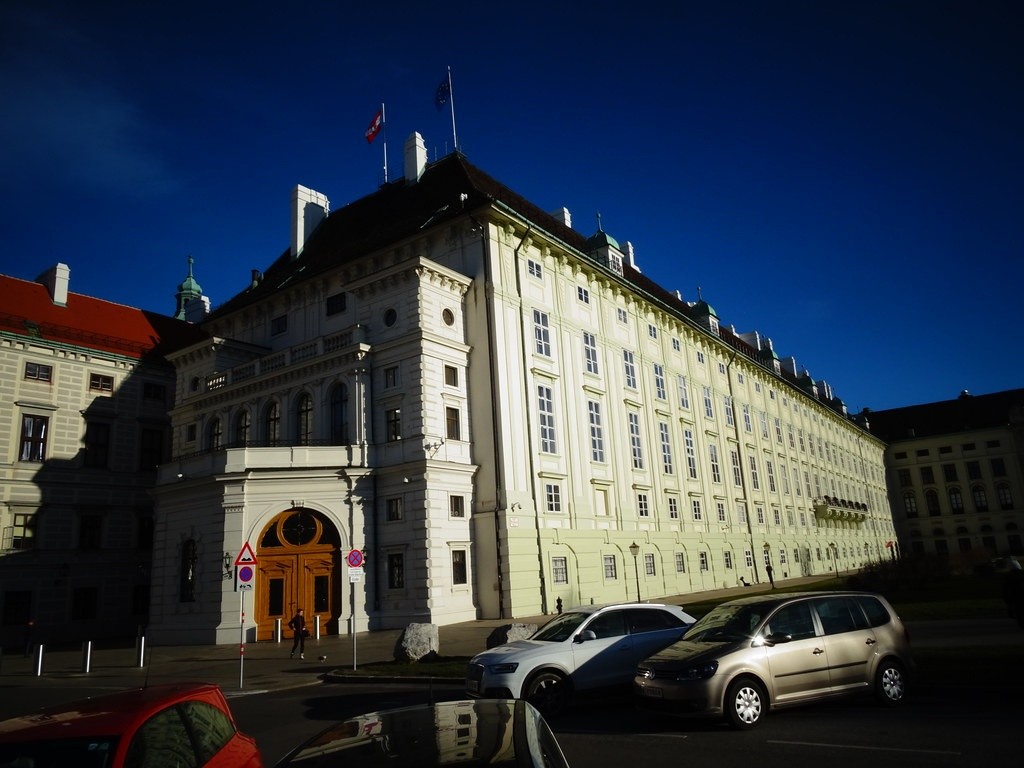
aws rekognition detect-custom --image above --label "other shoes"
[301,656,304,660]
[291,653,293,659]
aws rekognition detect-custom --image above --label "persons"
[288,609,307,660]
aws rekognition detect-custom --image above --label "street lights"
[864,542,870,565]
[829,542,838,577]
[629,539,640,602]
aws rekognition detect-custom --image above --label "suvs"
[634,589,909,729]
[462,603,697,714]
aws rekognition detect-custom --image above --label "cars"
[270,699,571,768]
[56,680,264,768]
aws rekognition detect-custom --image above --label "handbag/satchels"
[302,627,309,637]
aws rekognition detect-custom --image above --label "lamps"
[223,552,233,571]
[361,545,370,562]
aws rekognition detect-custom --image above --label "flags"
[364,111,382,144]
[436,74,450,112]
